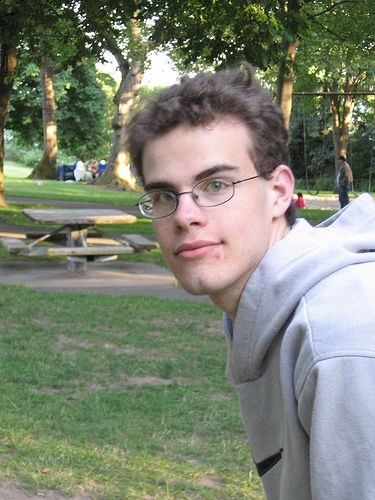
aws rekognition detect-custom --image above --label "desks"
[22,209,138,272]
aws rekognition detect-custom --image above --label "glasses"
[134,168,273,219]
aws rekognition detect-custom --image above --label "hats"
[337,156,345,160]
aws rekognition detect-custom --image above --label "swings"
[302,92,326,197]
[349,92,374,197]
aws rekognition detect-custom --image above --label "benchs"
[0,232,158,264]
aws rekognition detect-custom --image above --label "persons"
[86,160,107,180]
[337,156,352,208]
[294,193,305,208]
[121,62,375,500]
